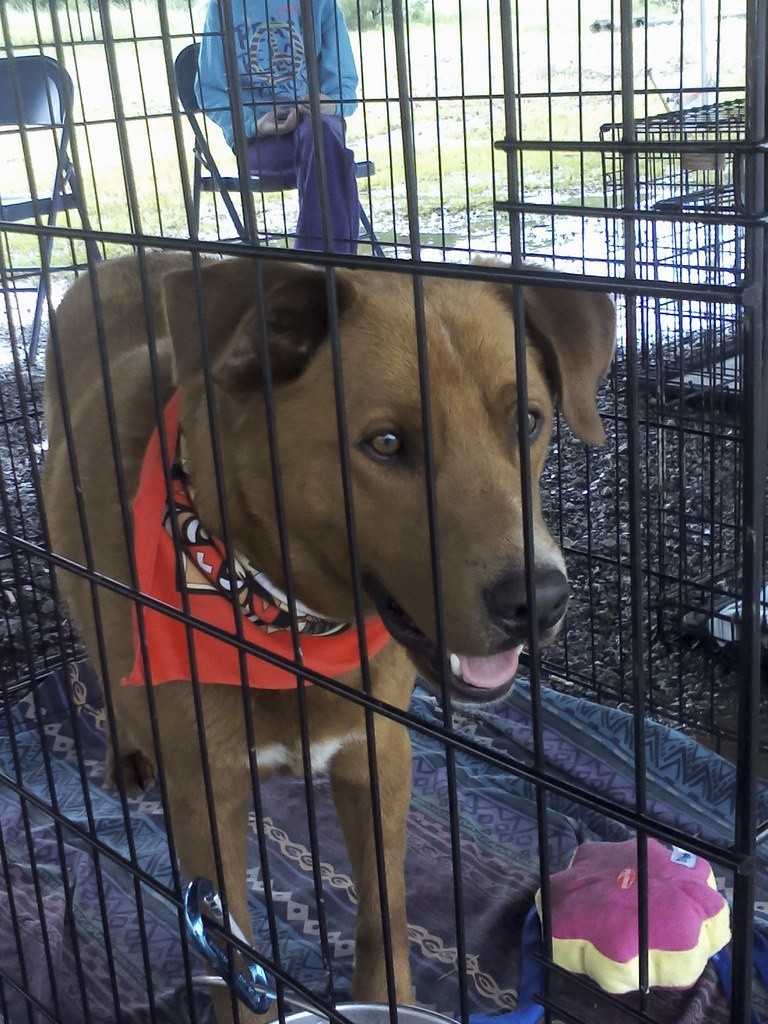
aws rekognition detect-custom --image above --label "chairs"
[174,41,388,258]
[0,55,105,365]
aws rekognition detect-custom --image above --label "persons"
[193,0,359,255]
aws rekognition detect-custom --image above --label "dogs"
[39,250,617,1023]
